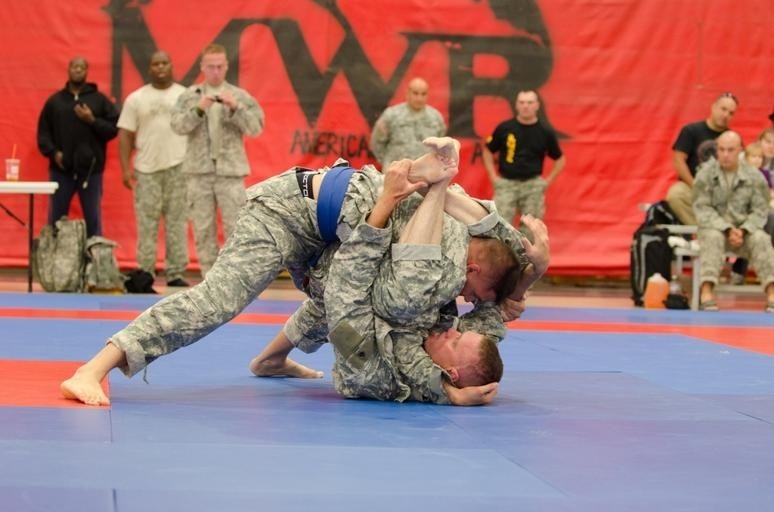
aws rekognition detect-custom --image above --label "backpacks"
[631,200,693,309]
[29,215,129,292]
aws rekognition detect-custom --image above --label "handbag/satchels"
[125,268,157,293]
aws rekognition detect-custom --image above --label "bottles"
[644,272,669,308]
[669,275,682,295]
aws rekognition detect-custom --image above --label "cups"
[4,160,20,180]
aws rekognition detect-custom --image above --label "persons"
[321,135,552,408]
[59,157,523,408]
[665,91,740,236]
[756,127,773,247]
[169,42,263,282]
[744,143,773,212]
[369,75,447,175]
[36,55,120,239]
[690,130,774,314]
[483,87,566,304]
[115,50,194,288]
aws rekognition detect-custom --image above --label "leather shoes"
[167,277,189,287]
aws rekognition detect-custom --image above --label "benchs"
[640,203,767,312]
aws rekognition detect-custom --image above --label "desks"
[1,181,60,291]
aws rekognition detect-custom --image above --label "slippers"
[698,300,719,311]
[764,302,774,313]
